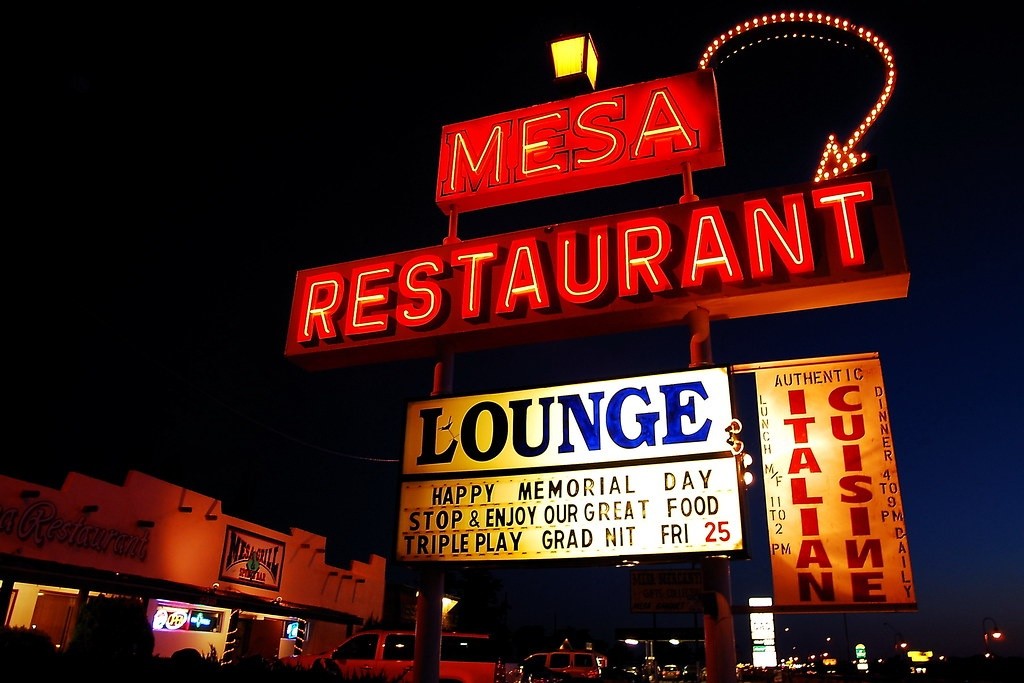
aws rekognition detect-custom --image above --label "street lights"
[982,617,1002,654]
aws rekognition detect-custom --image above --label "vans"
[521,651,601,680]
[282,632,496,683]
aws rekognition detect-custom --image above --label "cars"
[662,664,680,681]
